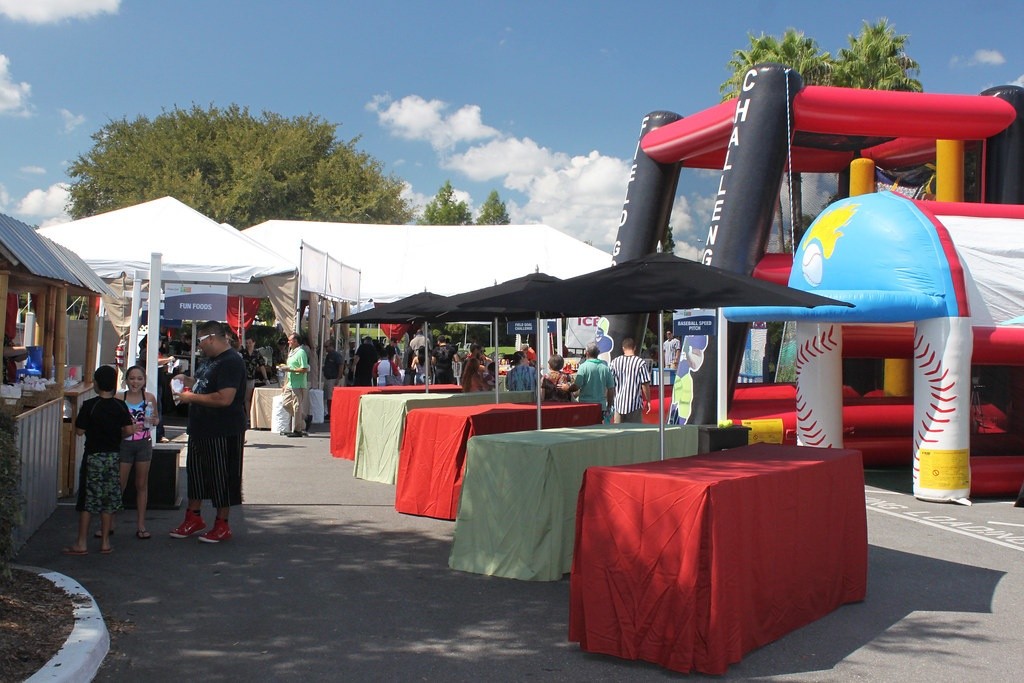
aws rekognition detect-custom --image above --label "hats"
[349,338,355,343]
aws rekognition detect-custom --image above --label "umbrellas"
[334,291,493,394]
[457,253,852,461]
[385,272,677,431]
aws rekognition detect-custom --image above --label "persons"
[277,332,313,437]
[321,340,344,423]
[557,342,615,424]
[540,355,579,404]
[608,338,651,423]
[506,351,539,391]
[660,329,681,370]
[519,344,536,367]
[62,365,134,554]
[237,336,271,427]
[95,366,159,539]
[484,362,499,392]
[137,327,494,389]
[172,320,247,544]
[3,335,31,387]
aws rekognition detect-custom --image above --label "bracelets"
[265,379,269,380]
[290,367,295,371]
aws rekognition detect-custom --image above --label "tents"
[234,220,614,302]
[32,195,299,446]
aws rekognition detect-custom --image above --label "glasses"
[197,333,215,343]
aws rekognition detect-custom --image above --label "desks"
[330,384,868,675]
[250,386,324,428]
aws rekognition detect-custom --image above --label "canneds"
[182,386,191,392]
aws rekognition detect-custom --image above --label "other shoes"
[304,414,313,432]
[287,430,302,437]
[160,436,169,443]
[324,413,330,420]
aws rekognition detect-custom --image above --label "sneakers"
[198,528,232,542]
[169,517,206,538]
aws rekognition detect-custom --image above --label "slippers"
[135,529,151,540]
[94,529,113,538]
[63,547,88,555]
[100,547,113,553]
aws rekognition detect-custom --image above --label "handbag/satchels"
[385,362,403,386]
